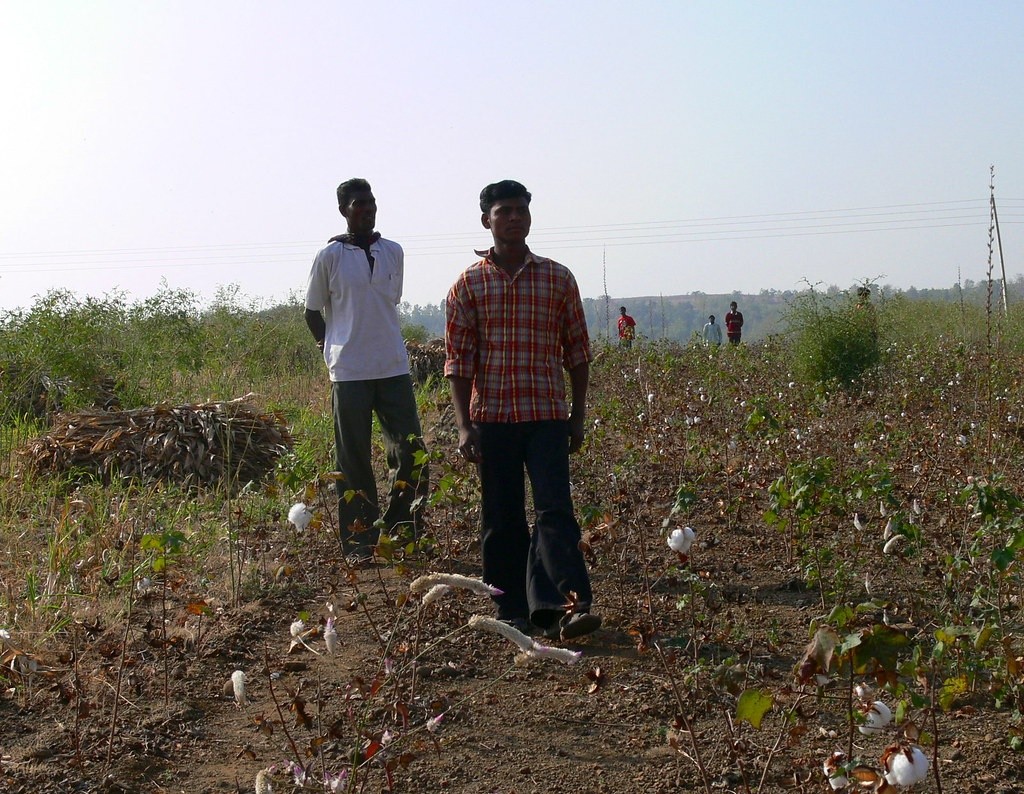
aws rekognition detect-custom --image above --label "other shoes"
[542,610,602,640]
[345,554,370,569]
[501,617,529,633]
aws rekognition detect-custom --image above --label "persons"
[701,315,723,349]
[302,177,429,566]
[446,181,603,639]
[725,301,744,345]
[855,287,879,344]
[617,307,635,348]
